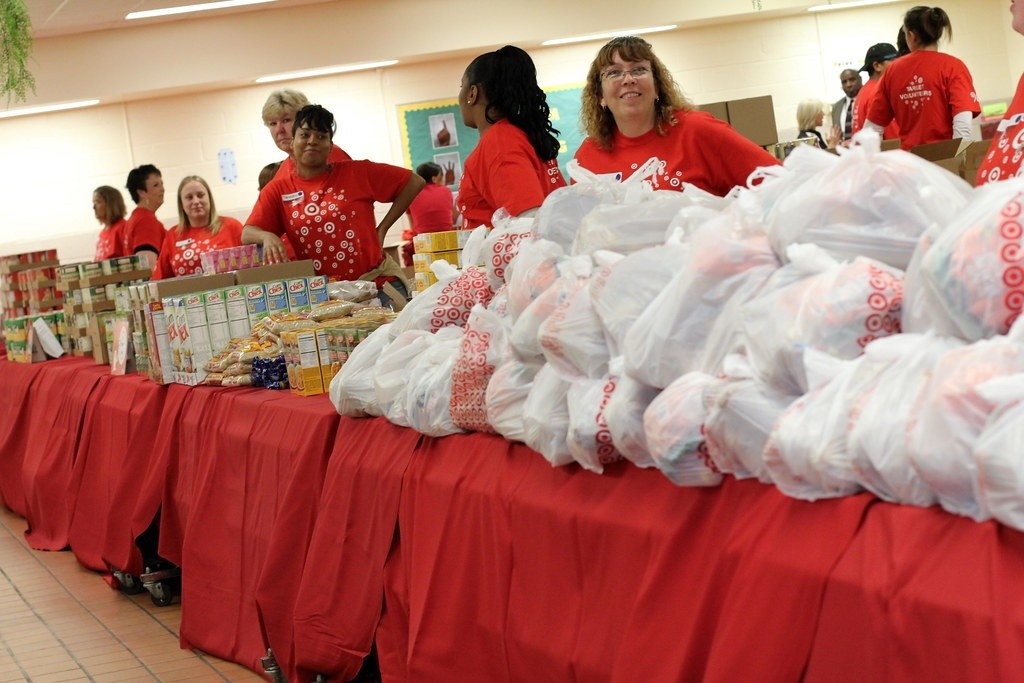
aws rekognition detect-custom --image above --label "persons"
[148,176,245,279]
[455,44,568,232]
[570,35,782,197]
[863,6,981,140]
[93,186,129,262]
[975,0,1024,185]
[240,105,425,309]
[406,163,454,236]
[830,69,863,144]
[120,164,168,270]
[795,98,842,150]
[257,160,296,264]
[261,89,352,164]
[852,24,912,142]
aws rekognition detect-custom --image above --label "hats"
[857,42,899,74]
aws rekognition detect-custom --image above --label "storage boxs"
[880,134,995,187]
[411,225,476,295]
[0,247,333,399]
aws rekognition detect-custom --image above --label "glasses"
[601,64,655,87]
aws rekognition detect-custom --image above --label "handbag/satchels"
[325,125,1024,536]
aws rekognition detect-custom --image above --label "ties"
[844,99,854,140]
